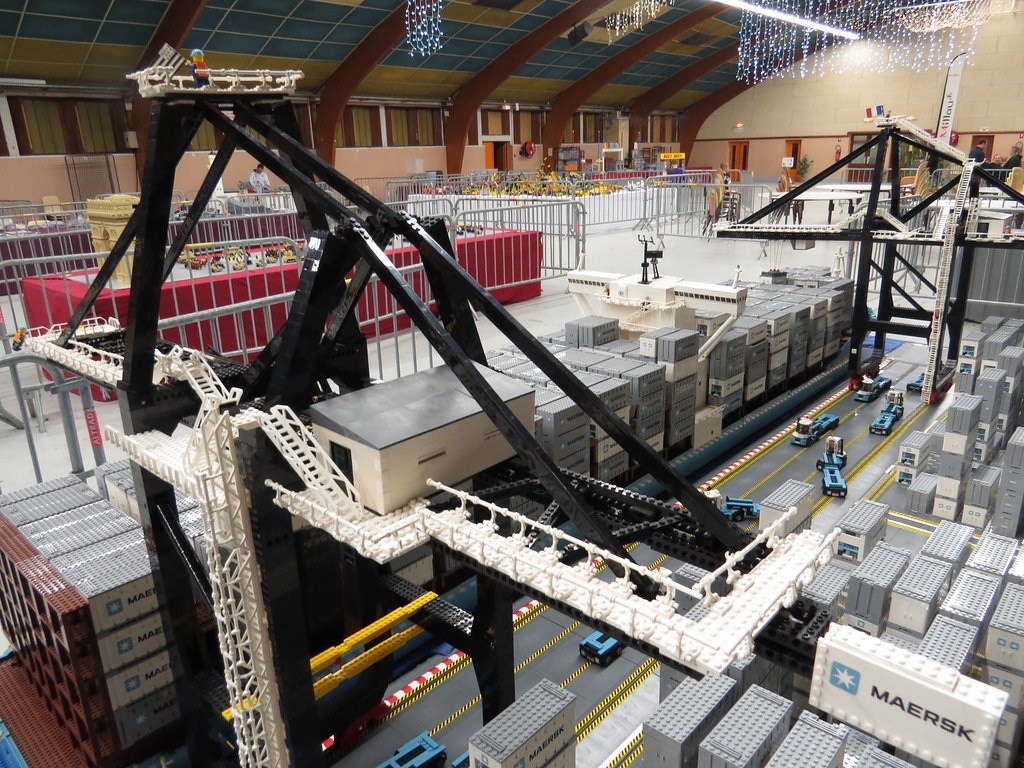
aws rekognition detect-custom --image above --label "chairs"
[701,167,741,243]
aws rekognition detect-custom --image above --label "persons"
[920,143,938,187]
[968,138,1023,208]
[667,159,688,188]
[249,164,270,204]
[721,162,732,215]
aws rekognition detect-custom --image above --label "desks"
[0,207,304,295]
[758,192,864,251]
[813,179,892,226]
[406,184,678,226]
[20,224,545,403]
